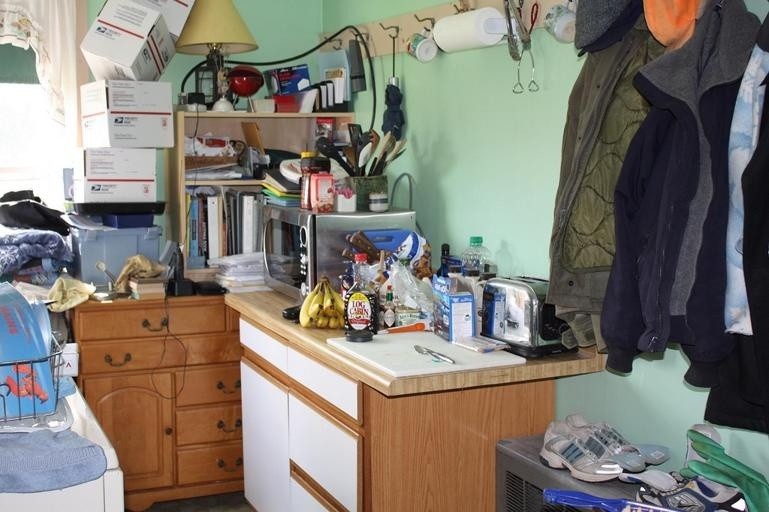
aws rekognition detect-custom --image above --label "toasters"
[475,274,580,362]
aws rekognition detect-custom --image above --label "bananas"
[299,276,346,329]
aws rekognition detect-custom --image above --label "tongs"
[501,0,531,62]
[365,128,406,177]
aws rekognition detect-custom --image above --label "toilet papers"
[433,7,507,52]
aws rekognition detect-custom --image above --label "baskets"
[0,332,63,422]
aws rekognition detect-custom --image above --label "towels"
[0,426,108,495]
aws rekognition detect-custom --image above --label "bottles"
[437,234,495,338]
[341,251,378,342]
[382,286,395,329]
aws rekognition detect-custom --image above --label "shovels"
[348,123,363,177]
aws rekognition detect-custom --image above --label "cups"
[348,174,388,213]
[334,192,357,214]
[542,0,579,44]
[402,23,438,65]
[368,191,388,213]
[396,306,421,328]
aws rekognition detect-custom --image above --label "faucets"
[95,262,117,285]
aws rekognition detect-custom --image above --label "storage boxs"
[72,148,158,203]
[66,212,164,294]
[79,0,177,87]
[130,0,196,46]
[79,79,175,149]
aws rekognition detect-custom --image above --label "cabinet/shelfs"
[225,288,607,511]
[170,109,356,284]
[62,288,245,512]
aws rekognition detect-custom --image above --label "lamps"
[175,1,262,113]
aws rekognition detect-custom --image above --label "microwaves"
[258,203,417,302]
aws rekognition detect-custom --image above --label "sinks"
[93,285,116,293]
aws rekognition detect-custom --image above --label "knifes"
[426,348,455,364]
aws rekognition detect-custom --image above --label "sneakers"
[539,414,749,512]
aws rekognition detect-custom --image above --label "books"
[186,170,301,295]
[129,275,165,301]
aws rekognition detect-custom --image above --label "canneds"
[301,157,332,211]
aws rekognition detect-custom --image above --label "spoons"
[412,344,441,362]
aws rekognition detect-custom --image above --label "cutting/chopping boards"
[325,327,527,378]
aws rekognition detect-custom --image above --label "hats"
[643,1,698,53]
[574,1,642,57]
